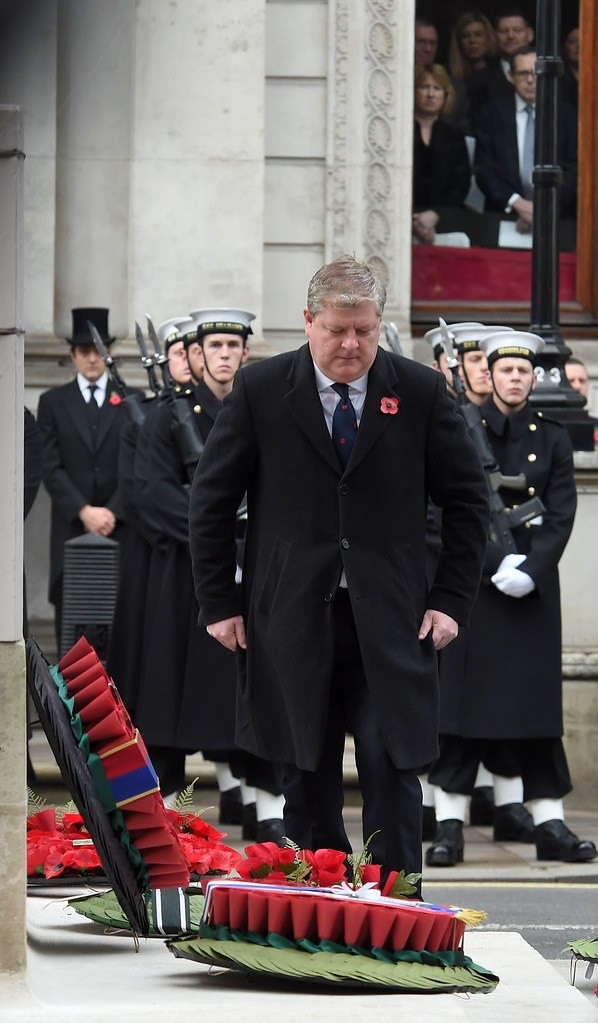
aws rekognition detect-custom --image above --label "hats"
[157,317,193,348]
[424,323,484,362]
[65,308,115,347]
[454,326,514,355]
[174,319,199,348]
[478,331,545,371]
[189,308,256,338]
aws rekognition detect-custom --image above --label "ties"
[85,385,101,446]
[330,382,358,474]
[523,105,535,191]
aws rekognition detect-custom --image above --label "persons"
[24,253,598,899]
[412,7,580,254]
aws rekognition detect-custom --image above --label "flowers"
[109,390,122,406]
[26,635,501,995]
[380,396,399,415]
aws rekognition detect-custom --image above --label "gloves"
[496,554,527,574]
[491,569,535,598]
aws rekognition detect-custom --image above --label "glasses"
[512,69,536,82]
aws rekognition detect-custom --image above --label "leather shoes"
[242,802,257,841]
[422,806,437,842]
[534,819,598,863]
[426,820,464,866]
[219,786,244,825]
[256,818,287,848]
[493,802,535,843]
[469,786,495,826]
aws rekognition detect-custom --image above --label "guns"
[145,314,203,485]
[439,316,547,556]
[134,321,163,394]
[88,319,146,430]
